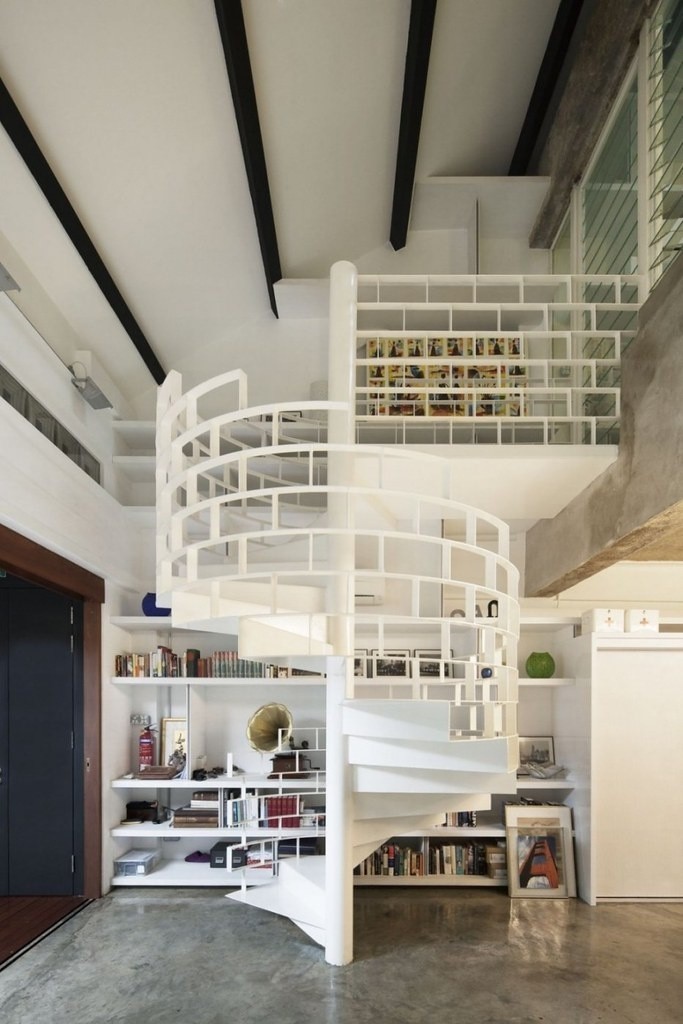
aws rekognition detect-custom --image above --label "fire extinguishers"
[137,724,158,772]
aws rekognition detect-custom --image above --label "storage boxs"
[113,847,162,875]
[581,608,625,634]
[624,610,660,633]
[210,840,248,867]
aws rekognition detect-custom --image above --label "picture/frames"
[504,805,577,899]
[366,339,531,416]
[161,717,188,767]
[415,649,454,677]
[354,649,368,677]
[516,736,555,775]
[261,410,302,424]
[371,649,411,678]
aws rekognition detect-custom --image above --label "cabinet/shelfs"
[112,613,574,886]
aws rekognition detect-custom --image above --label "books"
[354,837,508,881]
[173,789,325,828]
[441,811,478,828]
[114,645,288,677]
